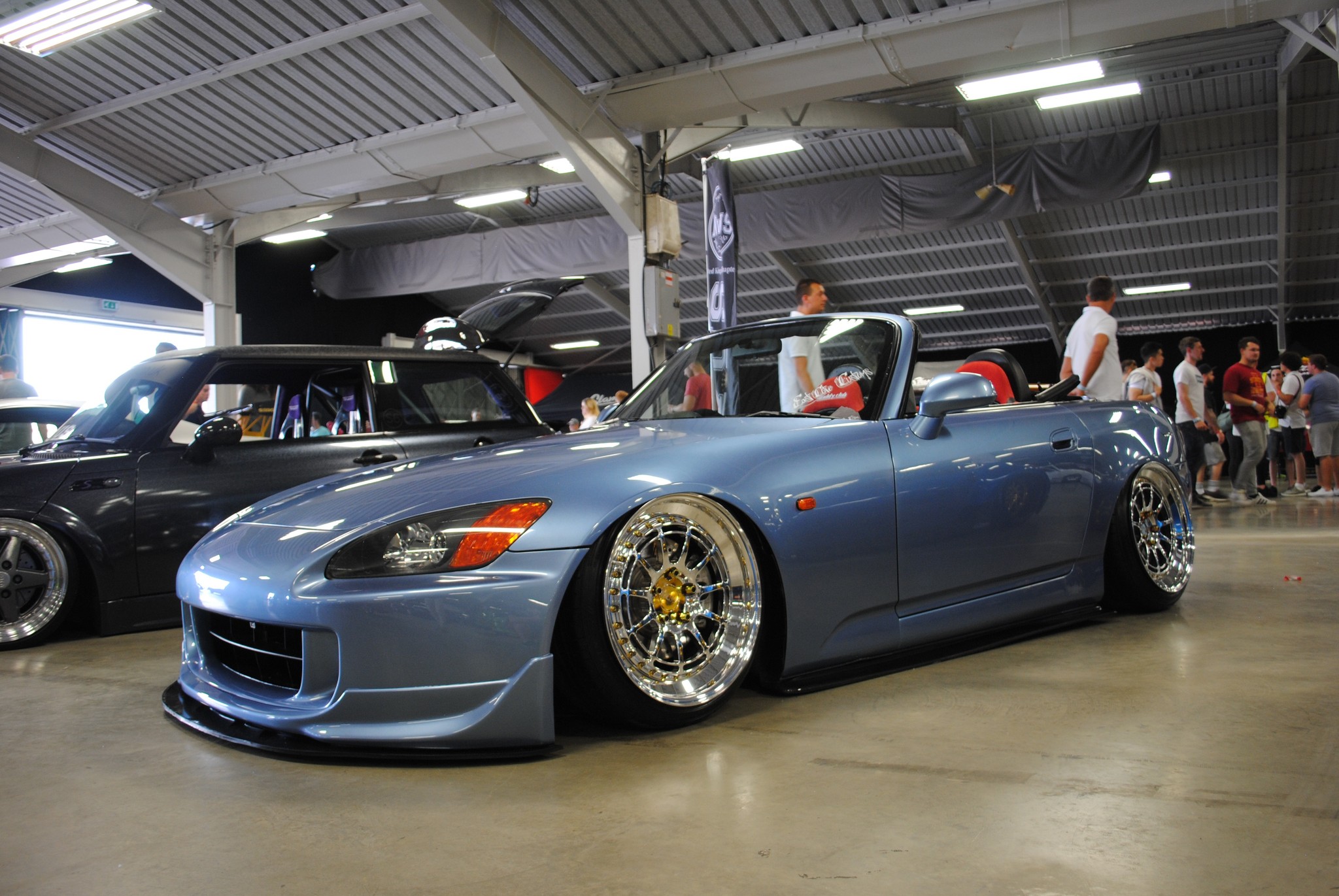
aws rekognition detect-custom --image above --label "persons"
[0,340,713,459]
[777,277,828,413]
[1123,335,1339,508]
[1059,275,1125,402]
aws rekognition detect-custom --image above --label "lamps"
[1122,281,1191,294]
[53,256,113,273]
[453,188,528,208]
[953,56,1107,101]
[1033,77,1141,110]
[261,229,328,244]
[537,156,575,174]
[902,304,963,316]
[711,138,804,162]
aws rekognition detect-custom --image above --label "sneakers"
[1236,490,1275,506]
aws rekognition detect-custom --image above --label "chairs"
[955,349,1031,405]
[330,383,362,435]
[802,363,875,416]
[278,394,304,439]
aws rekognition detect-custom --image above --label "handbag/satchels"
[1274,405,1287,418]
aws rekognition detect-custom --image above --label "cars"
[0,275,609,652]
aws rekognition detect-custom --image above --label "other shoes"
[1331,487,1339,495]
[1281,484,1307,496]
[1257,485,1278,497]
[1306,485,1322,492]
[1204,489,1230,501]
[1307,484,1333,496]
[1191,492,1212,508]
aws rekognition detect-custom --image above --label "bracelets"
[1193,417,1201,423]
[1277,390,1282,395]
[1216,430,1223,433]
[1251,401,1257,408]
[1076,384,1086,391]
[1152,392,1157,400]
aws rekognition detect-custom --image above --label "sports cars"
[159,310,1196,767]
